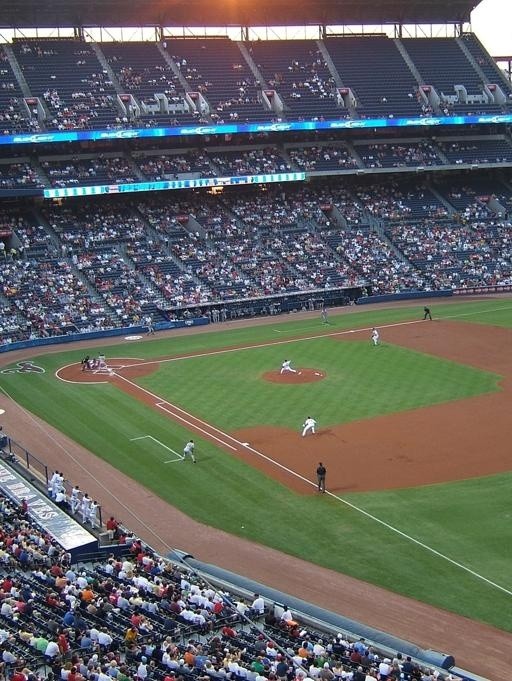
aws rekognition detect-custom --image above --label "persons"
[79,355,91,371]
[279,360,298,374]
[92,352,114,375]
[301,416,317,437]
[89,357,100,369]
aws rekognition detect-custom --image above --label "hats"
[0,491,464,681]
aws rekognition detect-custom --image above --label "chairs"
[208,603,314,681]
[0,567,260,681]
[294,630,385,681]
